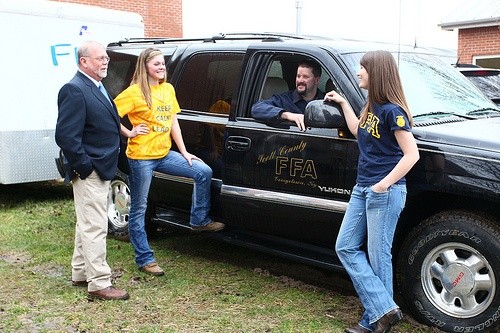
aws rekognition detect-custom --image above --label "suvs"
[101,32,500,333]
[448,43,500,110]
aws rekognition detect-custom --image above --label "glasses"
[82,56,110,62]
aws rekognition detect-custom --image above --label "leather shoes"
[87,287,129,300]
[72,280,86,285]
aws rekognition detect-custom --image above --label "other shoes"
[191,223,224,232]
[375,307,403,333]
[345,324,371,333]
[140,263,164,275]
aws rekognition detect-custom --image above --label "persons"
[55,41,129,300]
[323,50,421,333]
[251,59,326,132]
[115,48,226,275]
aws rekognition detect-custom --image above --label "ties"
[99,84,110,102]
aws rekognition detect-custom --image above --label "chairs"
[262,76,289,99]
